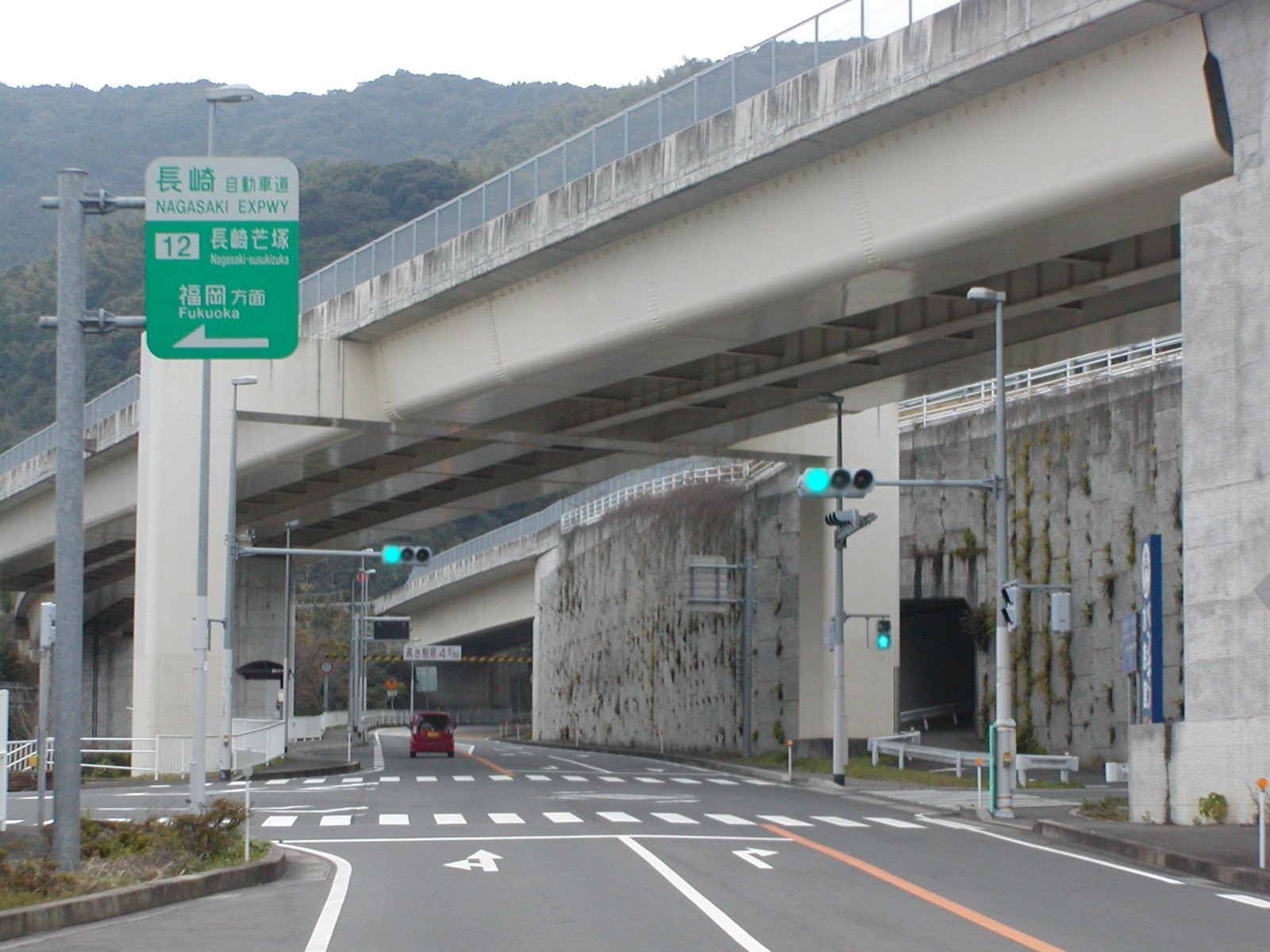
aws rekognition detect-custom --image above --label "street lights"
[347,568,366,743]
[219,374,260,783]
[516,647,527,723]
[190,85,257,819]
[357,547,375,738]
[281,521,301,753]
[966,286,1017,818]
[362,568,377,717]
[409,637,422,721]
[815,392,848,789]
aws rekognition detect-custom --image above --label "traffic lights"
[797,466,875,499]
[1001,585,1022,632]
[822,620,835,651]
[382,543,432,568]
[876,620,892,650]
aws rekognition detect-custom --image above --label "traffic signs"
[144,155,301,362]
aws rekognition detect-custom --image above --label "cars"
[406,711,457,757]
